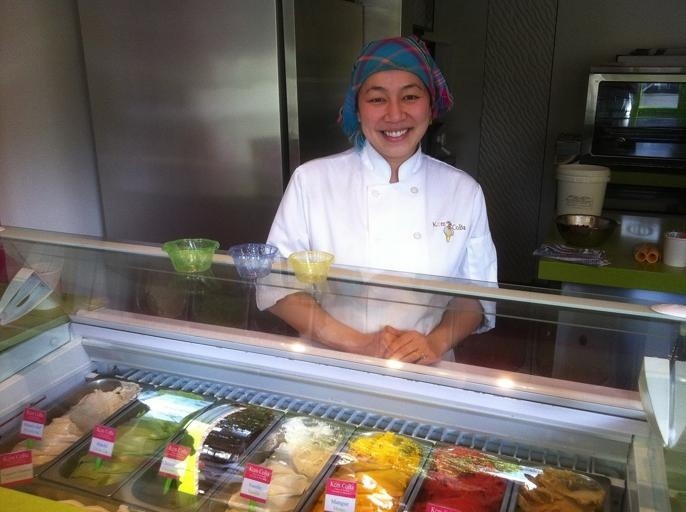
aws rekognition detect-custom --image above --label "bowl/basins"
[554,213,618,250]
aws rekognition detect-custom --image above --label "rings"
[422,352,427,360]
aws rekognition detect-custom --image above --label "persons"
[253,36,500,365]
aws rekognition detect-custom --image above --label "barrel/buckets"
[557,163,611,216]
